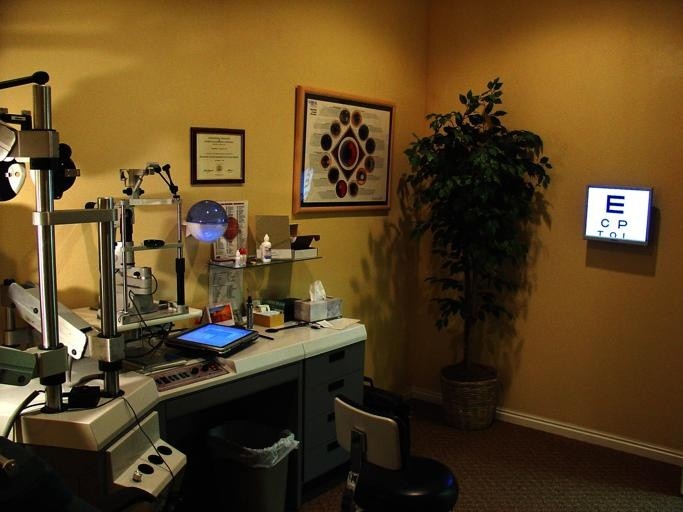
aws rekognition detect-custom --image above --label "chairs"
[332,393,460,510]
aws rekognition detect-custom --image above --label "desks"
[0,313,367,503]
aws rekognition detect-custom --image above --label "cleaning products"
[261,234,272,264]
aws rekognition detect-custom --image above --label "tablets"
[167,323,258,354]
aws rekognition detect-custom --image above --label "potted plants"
[397,74,552,433]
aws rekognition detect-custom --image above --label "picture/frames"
[188,126,245,185]
[290,83,397,216]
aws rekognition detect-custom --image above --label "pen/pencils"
[259,334,274,340]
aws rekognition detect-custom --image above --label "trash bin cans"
[208,419,295,511]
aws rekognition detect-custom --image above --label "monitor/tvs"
[583,185,653,247]
